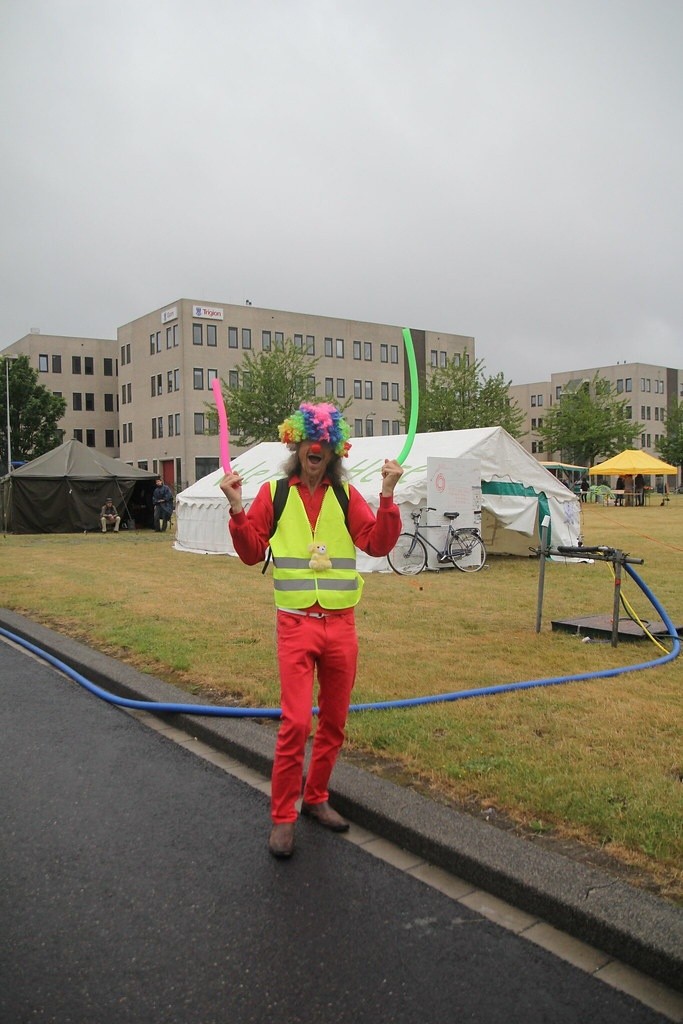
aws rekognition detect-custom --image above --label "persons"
[220,397,403,857]
[581,478,589,503]
[99,498,120,534]
[152,479,173,532]
[656,477,664,506]
[562,480,568,488]
[615,474,644,507]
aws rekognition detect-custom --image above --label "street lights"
[5,353,18,474]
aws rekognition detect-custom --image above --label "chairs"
[96,513,116,532]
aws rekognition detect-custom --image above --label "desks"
[575,492,597,503]
[603,493,642,506]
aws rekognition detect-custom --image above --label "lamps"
[164,451,168,454]
[245,299,252,306]
[616,361,620,364]
[624,360,627,363]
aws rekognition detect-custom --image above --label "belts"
[278,607,330,619]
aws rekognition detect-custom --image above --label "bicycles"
[387,506,487,575]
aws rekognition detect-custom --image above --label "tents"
[589,450,677,496]
[539,461,588,486]
[0,439,161,534]
[173,425,579,573]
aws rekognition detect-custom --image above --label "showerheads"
[557,542,613,553]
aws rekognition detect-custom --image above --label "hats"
[106,498,112,503]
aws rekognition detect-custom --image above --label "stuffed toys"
[309,544,332,572]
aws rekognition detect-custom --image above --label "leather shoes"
[269,822,296,856]
[300,800,350,832]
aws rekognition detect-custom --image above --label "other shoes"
[102,531,106,534]
[114,531,119,533]
